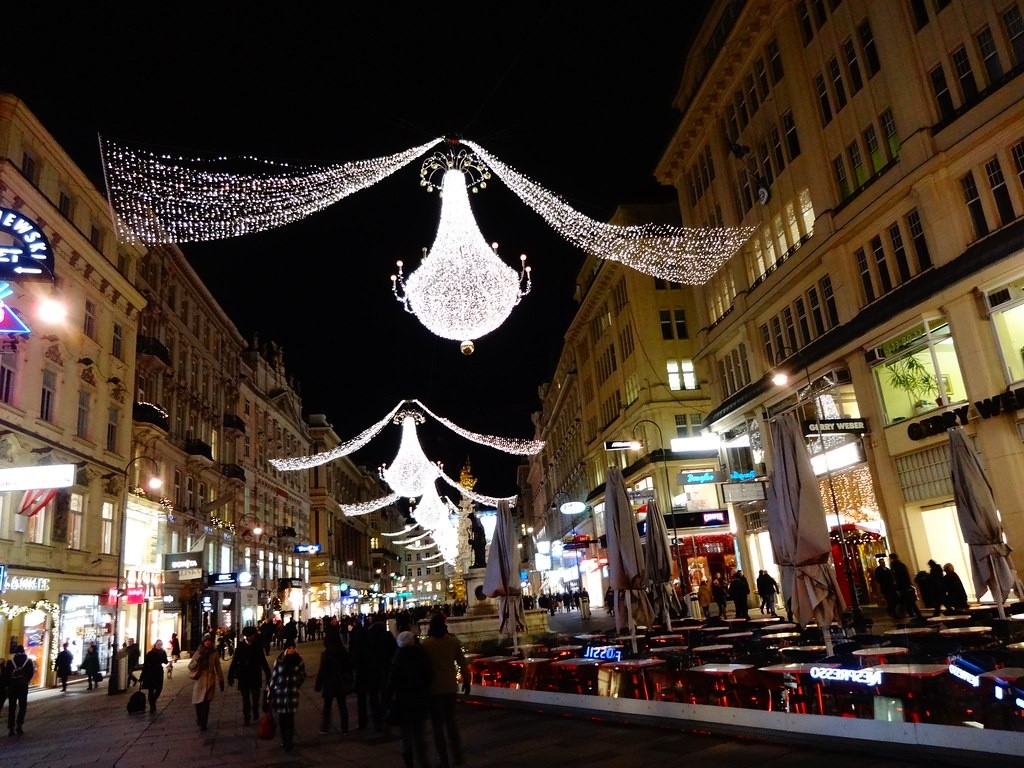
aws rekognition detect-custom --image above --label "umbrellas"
[605,466,656,654]
[769,415,838,657]
[644,499,674,631]
[482,501,528,655]
[947,426,1015,619]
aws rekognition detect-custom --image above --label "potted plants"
[887,357,951,406]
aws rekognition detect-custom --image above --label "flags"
[16,488,59,517]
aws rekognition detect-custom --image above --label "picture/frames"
[937,374,954,393]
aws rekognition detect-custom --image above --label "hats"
[201,636,212,643]
[396,631,415,647]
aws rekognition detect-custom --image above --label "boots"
[87,683,92,690]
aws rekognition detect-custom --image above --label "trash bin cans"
[579,597,591,620]
[686,592,702,618]
[116,652,129,692]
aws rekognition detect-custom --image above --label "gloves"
[461,683,471,696]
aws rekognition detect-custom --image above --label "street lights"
[107,456,162,695]
[772,347,864,616]
[340,558,353,620]
[550,491,582,594]
[234,512,262,646]
[631,420,686,594]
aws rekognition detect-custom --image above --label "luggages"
[127,681,146,714]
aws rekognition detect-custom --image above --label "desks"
[458,597,1023,729]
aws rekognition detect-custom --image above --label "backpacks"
[9,657,30,684]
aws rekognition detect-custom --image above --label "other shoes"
[196,720,208,731]
[244,713,251,724]
[453,758,465,768]
[62,689,65,691]
[133,679,138,685]
[341,727,348,736]
[15,723,24,734]
[318,729,328,735]
[355,723,368,729]
[435,762,450,768]
[9,727,14,736]
[254,711,259,720]
[280,743,293,754]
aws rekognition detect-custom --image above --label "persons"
[875,553,922,617]
[260,616,298,655]
[699,570,751,620]
[605,586,614,617]
[228,626,272,726]
[384,616,470,768]
[170,633,180,663]
[126,638,140,688]
[0,645,33,736]
[928,560,967,617]
[307,603,466,641]
[189,634,224,730]
[523,587,589,616]
[54,643,73,692]
[757,570,780,617]
[85,645,100,690]
[208,626,226,658]
[314,615,398,736]
[267,640,307,751]
[140,642,168,712]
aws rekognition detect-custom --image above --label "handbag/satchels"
[188,654,202,680]
[345,671,356,693]
[93,672,104,682]
[256,710,278,740]
[234,646,242,679]
